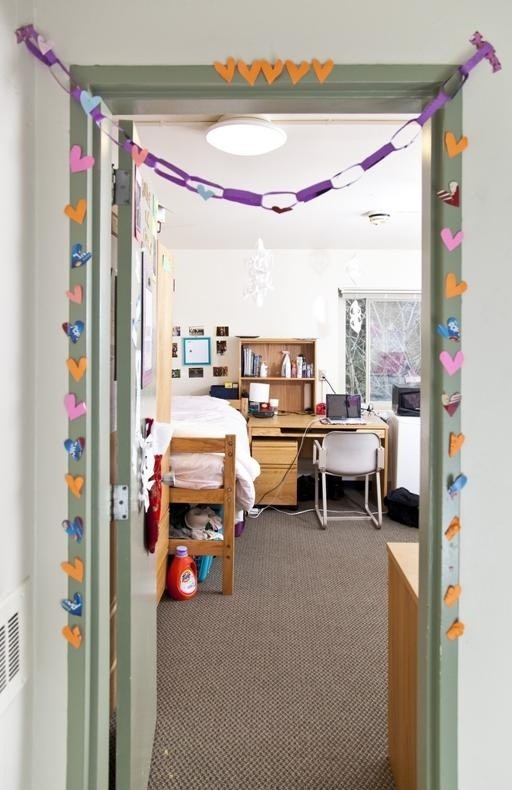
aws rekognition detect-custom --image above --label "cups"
[269,399,278,415]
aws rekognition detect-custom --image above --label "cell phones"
[320,419,327,424]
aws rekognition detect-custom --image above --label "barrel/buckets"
[249,383,271,403]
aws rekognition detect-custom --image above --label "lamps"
[248,382,269,416]
[204,114,287,157]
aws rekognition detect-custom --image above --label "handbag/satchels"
[383,487,419,528]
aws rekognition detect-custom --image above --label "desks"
[248,408,389,513]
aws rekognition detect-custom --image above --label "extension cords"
[245,508,259,515]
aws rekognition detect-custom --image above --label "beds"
[171,398,261,595]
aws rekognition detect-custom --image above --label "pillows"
[171,395,231,420]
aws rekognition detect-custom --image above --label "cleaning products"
[259,361,268,376]
[280,349,292,378]
[168,546,199,600]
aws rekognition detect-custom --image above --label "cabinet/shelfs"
[387,542,419,790]
[238,337,318,416]
[111,211,173,717]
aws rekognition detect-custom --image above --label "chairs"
[313,431,385,529]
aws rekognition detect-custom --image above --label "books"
[241,345,262,377]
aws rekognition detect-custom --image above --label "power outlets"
[320,375,327,380]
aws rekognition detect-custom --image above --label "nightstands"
[251,437,298,511]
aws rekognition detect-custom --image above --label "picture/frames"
[184,338,210,365]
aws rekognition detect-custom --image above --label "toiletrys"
[281,350,314,378]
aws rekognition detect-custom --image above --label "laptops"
[326,394,367,425]
